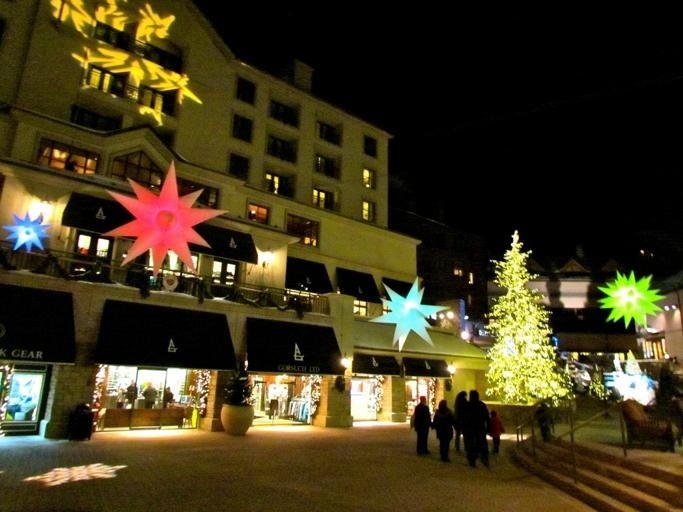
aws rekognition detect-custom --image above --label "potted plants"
[220,354,255,435]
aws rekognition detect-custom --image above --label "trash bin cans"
[64,404,94,441]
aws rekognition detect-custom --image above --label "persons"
[534,402,554,442]
[413,396,432,455]
[142,382,157,408]
[93,398,102,419]
[127,383,137,406]
[656,363,683,430]
[164,386,174,408]
[431,390,504,467]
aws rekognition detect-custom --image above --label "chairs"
[622,398,678,453]
